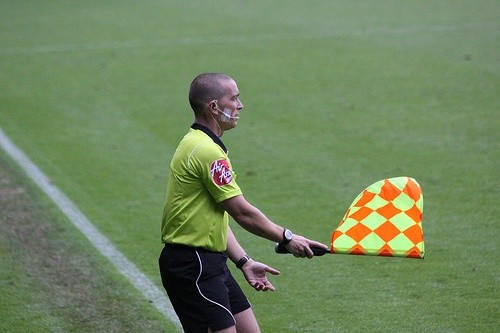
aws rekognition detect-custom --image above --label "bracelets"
[236,255,250,268]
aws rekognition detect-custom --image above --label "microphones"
[216,108,239,119]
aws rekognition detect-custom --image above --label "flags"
[330,177,425,258]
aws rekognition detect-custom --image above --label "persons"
[158,73,328,333]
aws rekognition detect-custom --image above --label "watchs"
[277,228,293,247]
